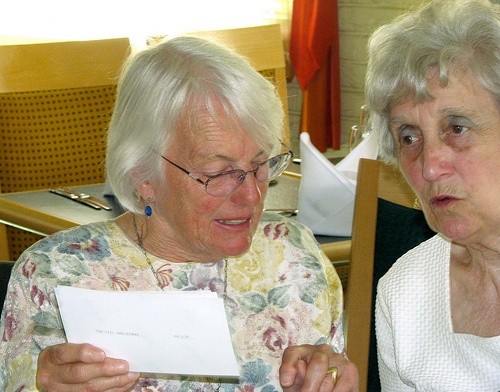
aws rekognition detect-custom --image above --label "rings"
[326,367,337,385]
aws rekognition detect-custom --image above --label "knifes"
[59,187,113,209]
[50,188,102,209]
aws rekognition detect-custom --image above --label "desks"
[0,155,351,262]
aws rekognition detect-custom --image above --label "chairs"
[145,23,291,157]
[0,38,133,261]
[347,158,437,392]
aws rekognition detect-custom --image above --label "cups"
[360,105,371,127]
[349,125,367,151]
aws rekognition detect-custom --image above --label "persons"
[365,0,499,392]
[0,38,362,391]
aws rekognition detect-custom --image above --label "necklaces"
[131,212,230,313]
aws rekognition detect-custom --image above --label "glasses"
[150,136,294,197]
